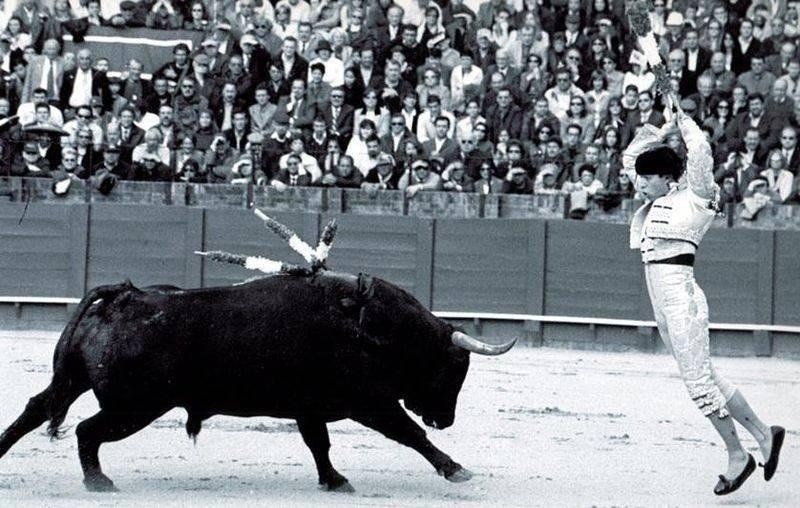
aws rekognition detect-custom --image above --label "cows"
[0,270,517,493]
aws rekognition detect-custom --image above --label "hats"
[635,147,680,175]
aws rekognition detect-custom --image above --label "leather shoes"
[758,425,785,482]
[713,453,756,496]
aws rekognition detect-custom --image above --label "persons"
[620,95,787,496]
[0,1,799,226]
